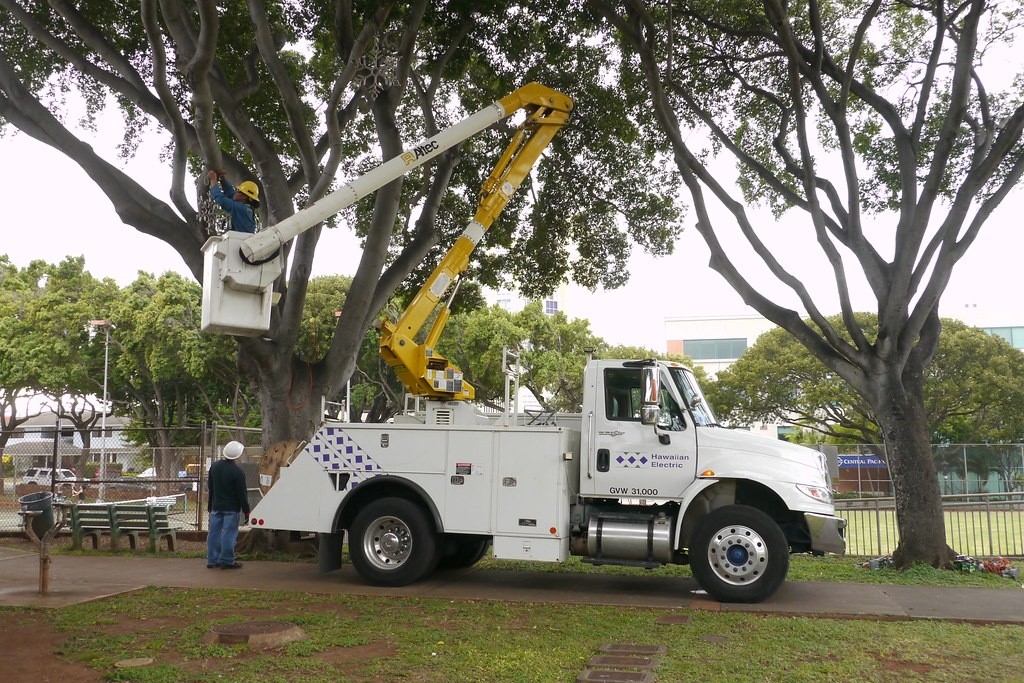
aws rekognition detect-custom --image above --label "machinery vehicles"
[196,82,850,603]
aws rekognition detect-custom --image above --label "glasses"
[236,188,249,198]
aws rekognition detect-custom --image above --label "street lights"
[95,324,116,504]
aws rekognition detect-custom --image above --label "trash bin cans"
[18,491,55,540]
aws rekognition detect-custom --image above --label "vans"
[22,466,77,494]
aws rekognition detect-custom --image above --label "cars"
[105,467,194,494]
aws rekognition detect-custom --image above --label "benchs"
[66,502,176,553]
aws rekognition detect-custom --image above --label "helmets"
[235,181,260,201]
[223,441,245,460]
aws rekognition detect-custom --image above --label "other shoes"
[207,562,222,568]
[222,562,243,569]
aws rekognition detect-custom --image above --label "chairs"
[609,396,619,418]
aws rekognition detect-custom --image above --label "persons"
[209,169,260,234]
[207,441,250,569]
